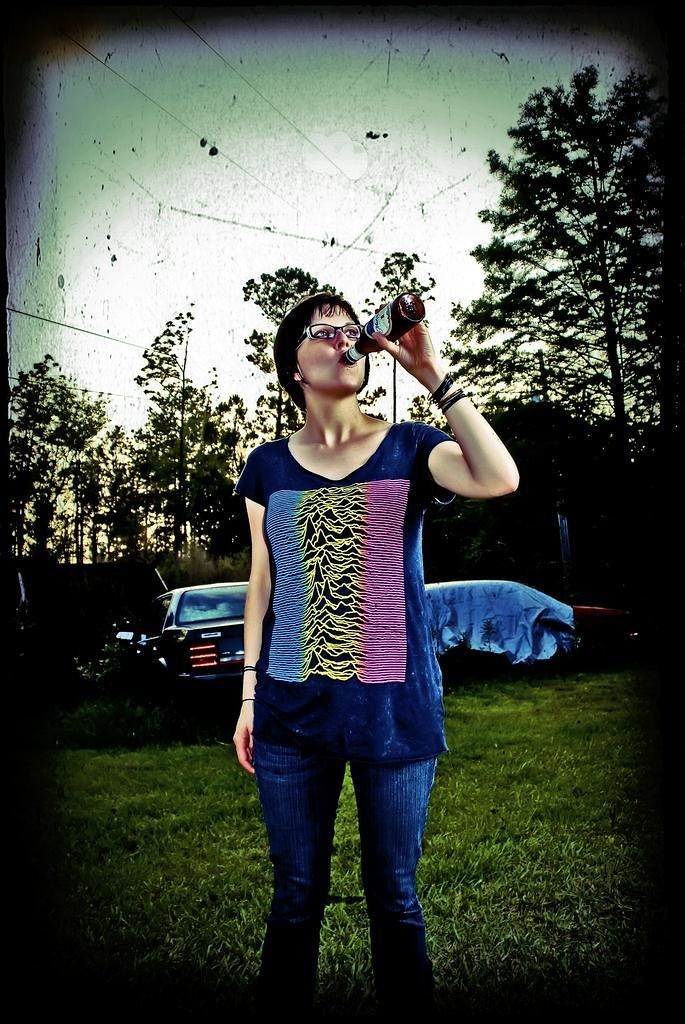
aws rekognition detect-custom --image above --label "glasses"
[295,324,365,352]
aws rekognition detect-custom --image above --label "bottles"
[342,293,425,365]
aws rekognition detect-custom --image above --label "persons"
[233,295,519,1024]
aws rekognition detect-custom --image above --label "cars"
[116,581,249,692]
[553,602,642,666]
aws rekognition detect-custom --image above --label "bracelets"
[436,388,467,414]
[242,666,255,671]
[241,699,254,702]
[427,373,455,404]
